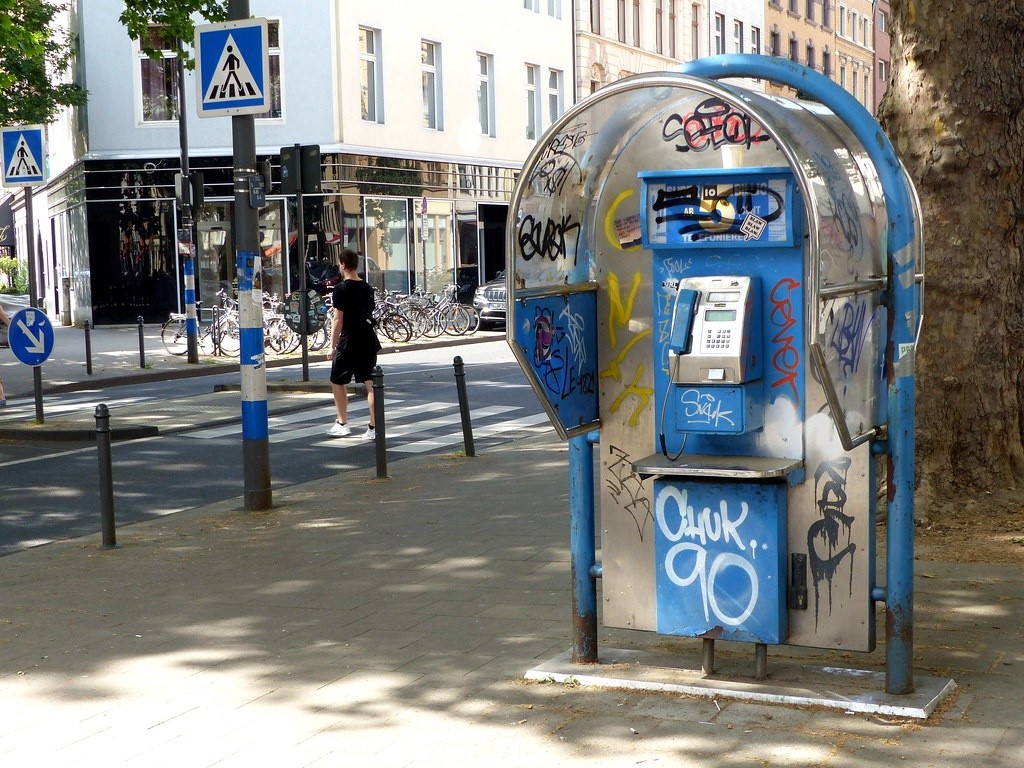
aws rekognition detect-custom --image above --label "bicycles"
[161,285,480,360]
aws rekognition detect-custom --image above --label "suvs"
[473,267,507,331]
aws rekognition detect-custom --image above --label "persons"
[0,305,11,408]
[326,248,382,440]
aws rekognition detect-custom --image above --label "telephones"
[667,275,764,388]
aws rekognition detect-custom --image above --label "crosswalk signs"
[0,124,48,188]
[193,18,270,118]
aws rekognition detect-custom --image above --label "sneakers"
[364,424,377,440]
[326,420,352,437]
